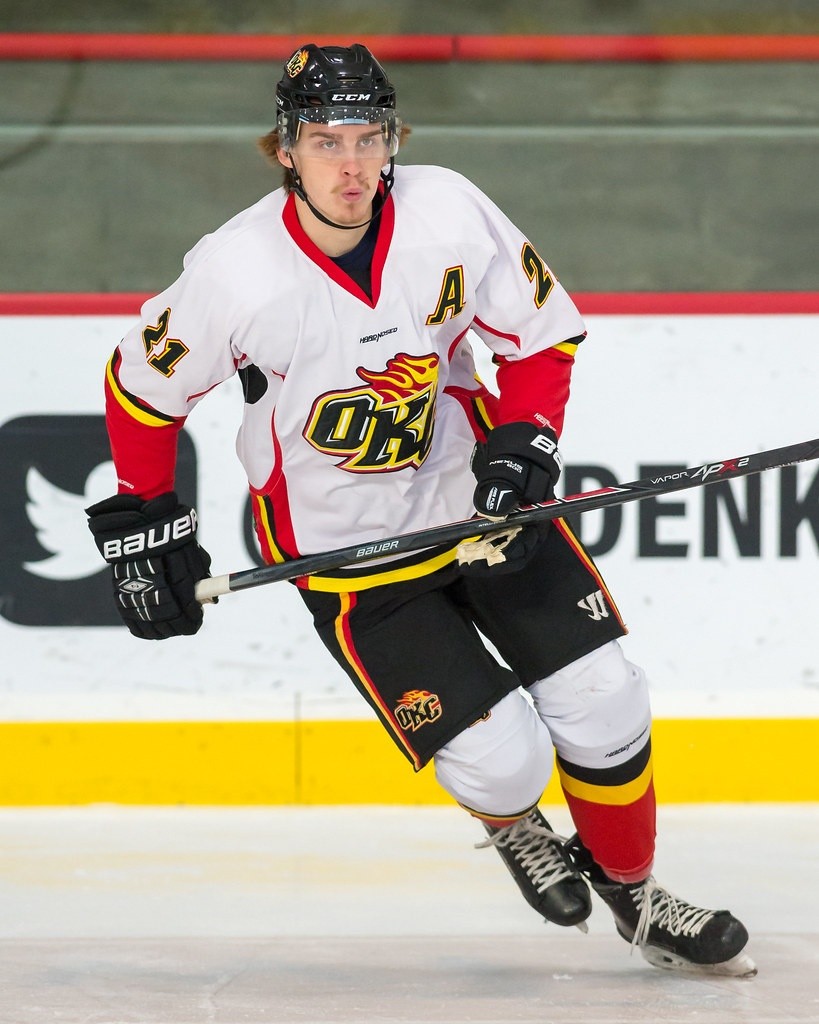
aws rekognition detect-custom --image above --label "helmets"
[275,43,396,140]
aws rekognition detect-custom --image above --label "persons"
[86,44,758,978]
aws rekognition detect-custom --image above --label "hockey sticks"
[194,438,819,603]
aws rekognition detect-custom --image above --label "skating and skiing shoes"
[563,832,758,978]
[483,805,592,934]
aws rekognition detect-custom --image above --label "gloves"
[457,422,563,582]
[84,493,219,640]
